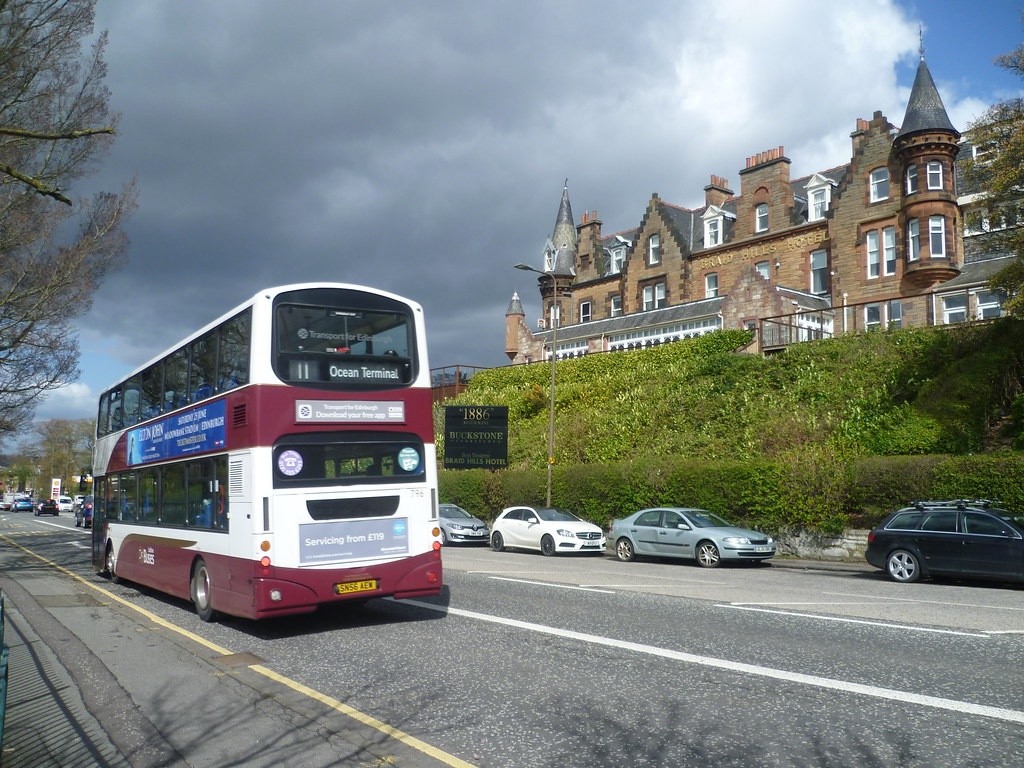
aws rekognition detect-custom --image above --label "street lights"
[512,262,557,508]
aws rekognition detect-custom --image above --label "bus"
[92,282,442,622]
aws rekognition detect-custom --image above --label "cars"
[0,499,13,508]
[56,497,74,512]
[489,505,607,556]
[10,498,33,513]
[608,507,778,568]
[35,499,59,516]
[74,495,83,504]
[75,496,93,528]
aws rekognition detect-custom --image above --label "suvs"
[865,498,1024,583]
[439,504,491,546]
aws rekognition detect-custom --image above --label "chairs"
[196,502,213,529]
[112,370,237,432]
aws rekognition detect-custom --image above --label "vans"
[6,492,29,502]
